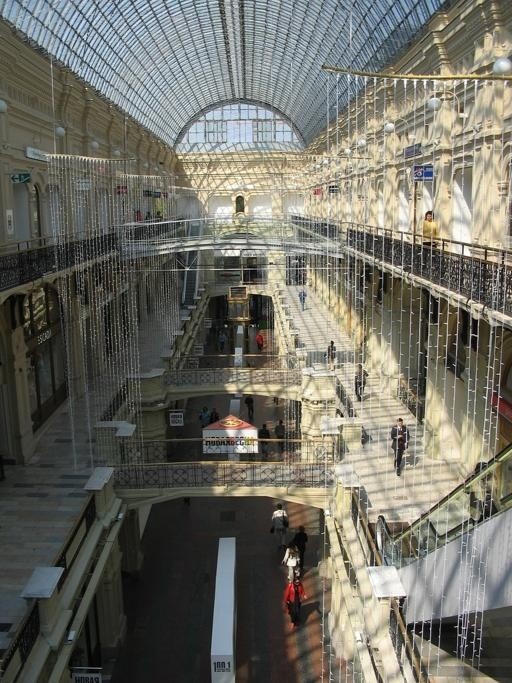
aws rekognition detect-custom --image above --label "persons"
[323,338,337,371]
[244,393,255,419]
[353,362,369,403]
[273,417,287,451]
[254,332,264,352]
[258,423,271,457]
[298,286,308,310]
[217,330,226,353]
[389,416,409,476]
[421,207,441,270]
[269,502,309,632]
[135,208,164,237]
[198,405,220,427]
[465,461,497,525]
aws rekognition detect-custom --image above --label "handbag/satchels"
[283,517,289,528]
[270,526,275,533]
